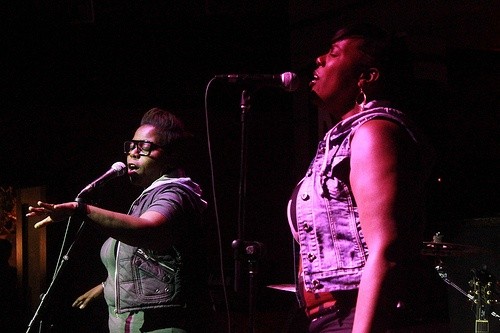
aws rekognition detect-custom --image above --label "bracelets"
[73,200,88,215]
[101,282,105,288]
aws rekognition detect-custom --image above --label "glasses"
[122,139,168,156]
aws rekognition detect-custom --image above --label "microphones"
[78,161,127,197]
[226,72,300,92]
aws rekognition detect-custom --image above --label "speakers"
[447,225,500,333]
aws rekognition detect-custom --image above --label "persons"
[25,105,222,333]
[282,19,428,333]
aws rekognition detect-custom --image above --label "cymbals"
[266,284,296,293]
[409,242,496,257]
